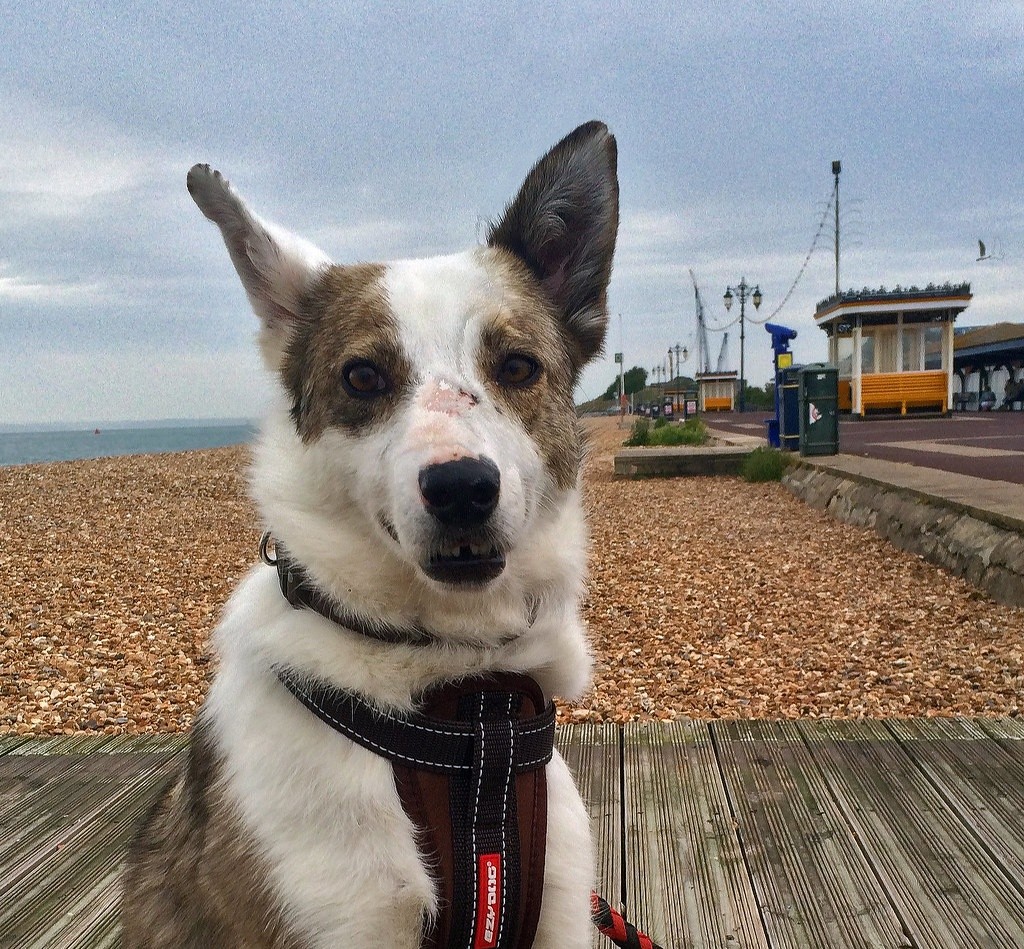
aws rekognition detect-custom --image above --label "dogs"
[122,121,622,949]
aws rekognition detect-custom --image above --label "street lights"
[724,275,763,413]
[668,344,689,413]
[652,364,666,404]
